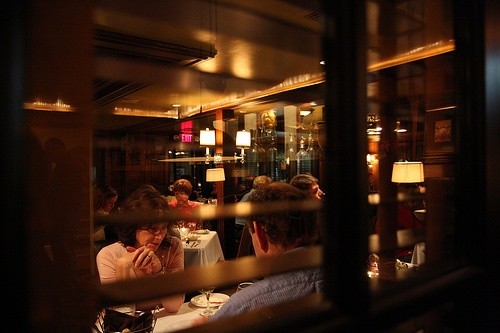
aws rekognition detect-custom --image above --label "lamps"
[200,128,219,164]
[219,130,251,163]
[206,168,225,182]
[391,161,424,193]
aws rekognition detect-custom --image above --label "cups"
[173,225,179,232]
[236,282,253,292]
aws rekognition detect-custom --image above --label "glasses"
[138,223,169,233]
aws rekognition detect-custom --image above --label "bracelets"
[159,267,166,273]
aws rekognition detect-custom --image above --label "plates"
[195,230,210,234]
[190,292,230,307]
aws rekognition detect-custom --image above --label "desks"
[152,301,218,333]
[182,230,224,270]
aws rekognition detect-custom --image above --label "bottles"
[247,127,321,186]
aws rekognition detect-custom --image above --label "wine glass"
[200,287,217,318]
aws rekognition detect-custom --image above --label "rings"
[147,255,151,258]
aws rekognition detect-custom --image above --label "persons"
[240,176,272,202]
[204,182,322,318]
[96,186,118,216]
[374,184,425,263]
[96,184,185,315]
[168,180,204,208]
[290,174,323,199]
